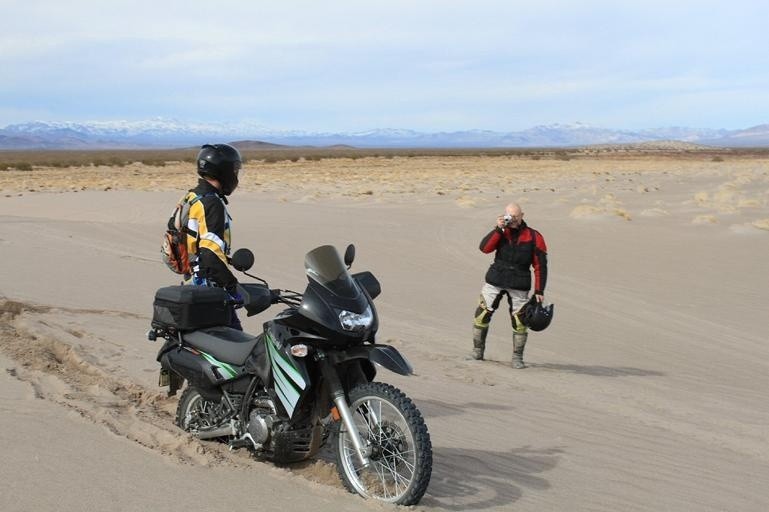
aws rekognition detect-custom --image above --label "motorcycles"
[144,244,435,507]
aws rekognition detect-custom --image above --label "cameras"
[504,216,512,226]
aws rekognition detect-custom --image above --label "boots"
[511,333,527,369]
[466,324,488,361]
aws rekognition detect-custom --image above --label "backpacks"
[159,192,227,275]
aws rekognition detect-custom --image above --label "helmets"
[525,301,553,331]
[197,143,243,195]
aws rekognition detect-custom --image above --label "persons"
[472,205,547,369]
[162,143,243,333]
